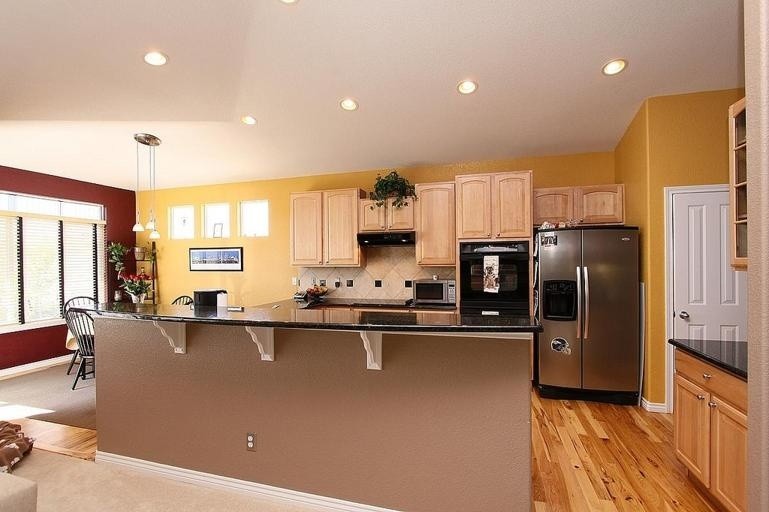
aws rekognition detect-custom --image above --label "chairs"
[64,295,194,391]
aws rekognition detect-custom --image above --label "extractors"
[358,232,414,246]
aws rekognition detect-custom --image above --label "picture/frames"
[189,247,243,271]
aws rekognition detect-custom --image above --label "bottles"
[113,289,122,301]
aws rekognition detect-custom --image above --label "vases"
[125,291,146,304]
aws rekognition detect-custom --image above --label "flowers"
[119,272,151,296]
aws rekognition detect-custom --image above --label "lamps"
[132,132,162,239]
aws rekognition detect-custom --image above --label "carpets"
[11,447,348,511]
[0,360,98,431]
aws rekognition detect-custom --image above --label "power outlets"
[291,277,341,287]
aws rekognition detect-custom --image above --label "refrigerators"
[537,227,640,407]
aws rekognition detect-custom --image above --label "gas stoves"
[352,299,409,308]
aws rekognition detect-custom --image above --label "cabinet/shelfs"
[728,96,748,272]
[290,187,368,269]
[109,241,155,305]
[532,183,625,228]
[673,346,748,512]
[455,169,534,241]
[359,196,417,232]
[415,181,457,267]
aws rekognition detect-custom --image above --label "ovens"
[460,242,531,307]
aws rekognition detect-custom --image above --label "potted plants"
[370,171,419,210]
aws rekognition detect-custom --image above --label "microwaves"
[412,280,456,303]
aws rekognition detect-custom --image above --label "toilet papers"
[216,292,228,307]
